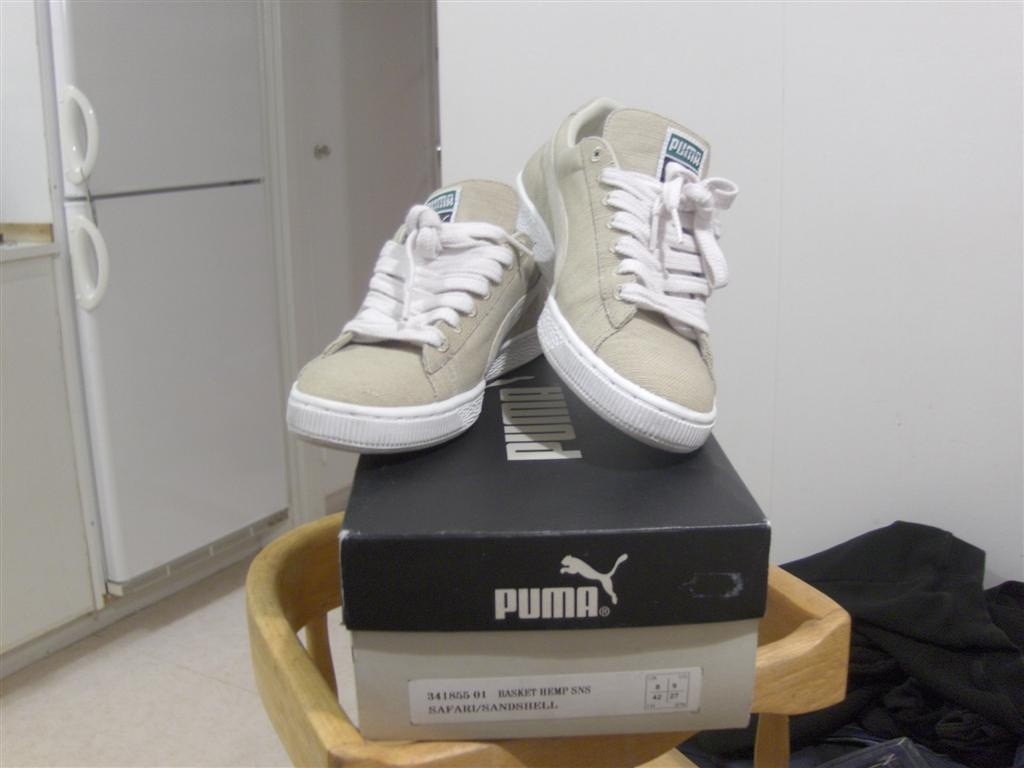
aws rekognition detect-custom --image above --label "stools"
[244,511,851,768]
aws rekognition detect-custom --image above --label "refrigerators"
[1,0,293,596]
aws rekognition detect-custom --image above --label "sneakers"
[287,178,552,452]
[517,98,739,452]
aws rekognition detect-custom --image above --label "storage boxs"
[338,353,771,740]
